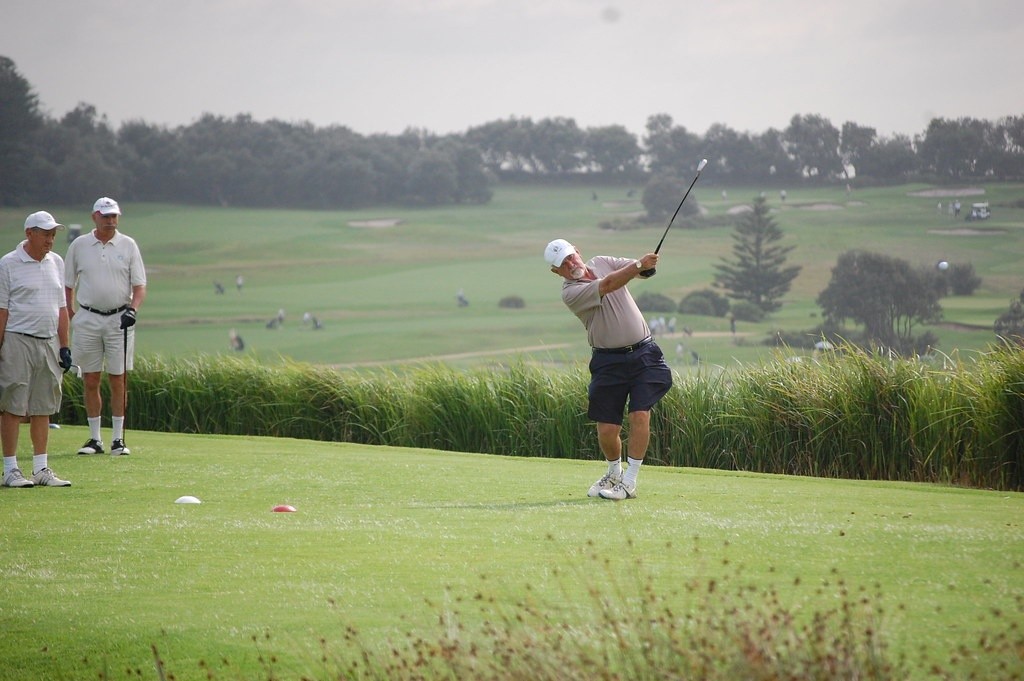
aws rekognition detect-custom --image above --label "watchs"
[634,260,643,272]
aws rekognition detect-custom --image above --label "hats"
[543,239,577,267]
[24,211,65,232]
[92,197,122,215]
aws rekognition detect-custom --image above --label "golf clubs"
[119,328,129,455]
[654,158,707,255]
[71,364,82,379]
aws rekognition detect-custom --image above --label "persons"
[227,329,243,355]
[542,239,672,500]
[64,197,146,456]
[937,200,962,218]
[965,202,991,221]
[235,273,244,292]
[303,311,321,328]
[675,341,686,367]
[266,309,285,329]
[645,313,679,334]
[0,210,72,487]
[210,279,224,294]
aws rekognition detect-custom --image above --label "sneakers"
[31,468,71,487]
[599,483,637,499]
[587,469,625,496]
[1,468,34,488]
[77,438,105,454]
[110,438,130,455]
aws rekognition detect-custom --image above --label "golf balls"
[938,262,949,270]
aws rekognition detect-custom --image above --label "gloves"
[58,347,72,373]
[639,267,657,278]
[120,308,136,329]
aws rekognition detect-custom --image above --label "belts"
[592,336,652,353]
[16,332,50,339]
[79,304,128,316]
